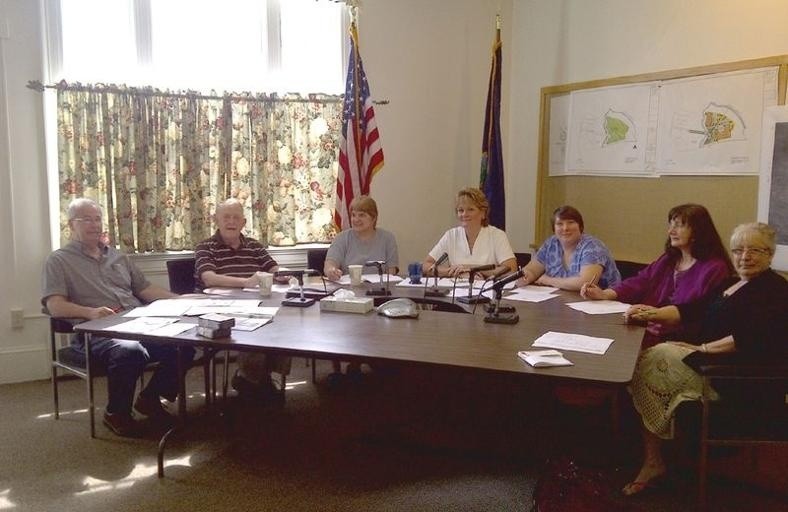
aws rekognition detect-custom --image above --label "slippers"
[623,479,665,495]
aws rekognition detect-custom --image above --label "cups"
[257,272,273,297]
[348,264,362,286]
[408,262,423,285]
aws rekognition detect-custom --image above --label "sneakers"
[103,409,139,436]
[135,394,169,420]
[232,368,284,402]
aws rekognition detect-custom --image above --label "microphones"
[429,251,448,271]
[365,261,385,267]
[469,263,496,273]
[274,269,317,277]
[480,270,525,293]
[487,266,511,282]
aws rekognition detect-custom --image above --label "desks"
[72,272,648,468]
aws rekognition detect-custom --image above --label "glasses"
[732,248,767,256]
[73,217,100,223]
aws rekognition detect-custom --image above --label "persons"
[580,203,736,350]
[623,222,788,498]
[422,186,518,280]
[517,205,623,291]
[323,195,399,385]
[193,198,291,396]
[40,198,196,439]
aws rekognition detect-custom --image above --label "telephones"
[376,298,420,319]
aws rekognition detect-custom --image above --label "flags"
[333,22,385,234]
[479,41,506,231]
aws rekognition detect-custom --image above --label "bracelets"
[701,343,707,354]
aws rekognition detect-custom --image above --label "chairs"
[306,247,343,380]
[49,317,148,437]
[165,257,303,403]
[700,360,787,505]
[514,252,532,272]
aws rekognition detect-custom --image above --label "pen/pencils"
[587,273,597,288]
[113,305,122,312]
